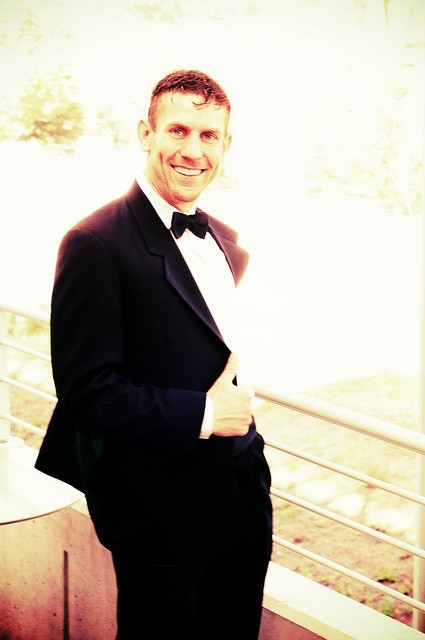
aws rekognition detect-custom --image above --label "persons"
[35,70,273,639]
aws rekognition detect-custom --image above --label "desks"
[0,445,85,525]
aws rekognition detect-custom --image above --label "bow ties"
[170,212,207,239]
